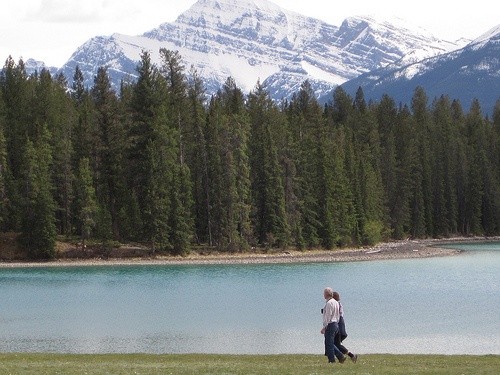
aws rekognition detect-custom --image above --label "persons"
[325,292,357,364]
[320,287,346,364]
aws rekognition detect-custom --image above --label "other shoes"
[339,355,346,363]
[352,354,357,363]
